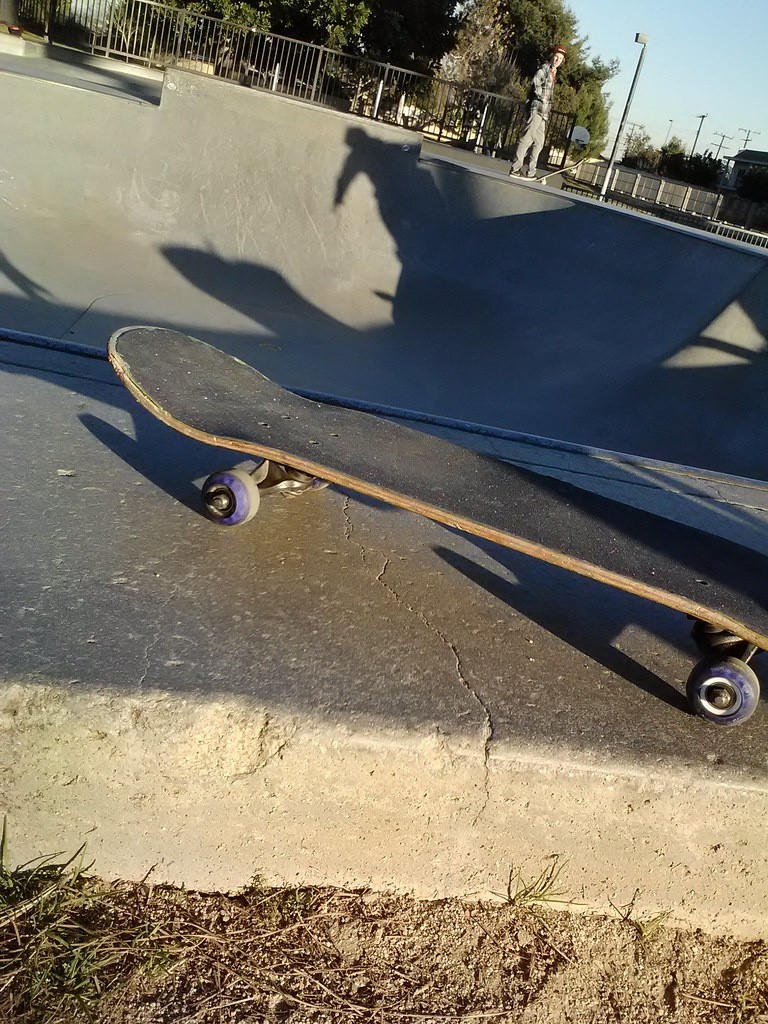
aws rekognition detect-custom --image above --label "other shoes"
[509,167,529,179]
[526,175,538,180]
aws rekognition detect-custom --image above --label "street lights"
[663,119,673,146]
[691,112,709,154]
[598,32,651,201]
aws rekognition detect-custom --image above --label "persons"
[509,45,568,182]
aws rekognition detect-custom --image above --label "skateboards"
[534,158,587,185]
[106,322,768,727]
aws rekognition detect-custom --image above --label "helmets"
[552,45,568,54]
